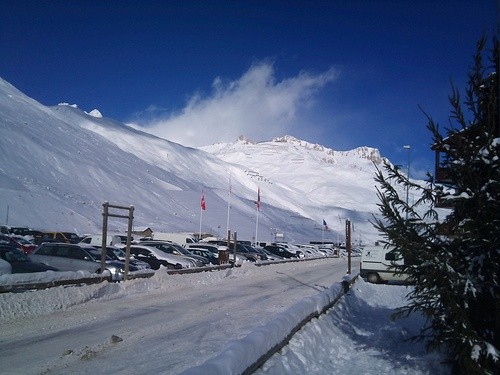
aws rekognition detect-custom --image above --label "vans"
[359,246,426,285]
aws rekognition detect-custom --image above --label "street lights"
[403,145,411,220]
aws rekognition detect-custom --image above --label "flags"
[322,219,329,232]
[201,190,207,210]
[256,189,261,210]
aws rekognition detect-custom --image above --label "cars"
[0,223,363,278]
